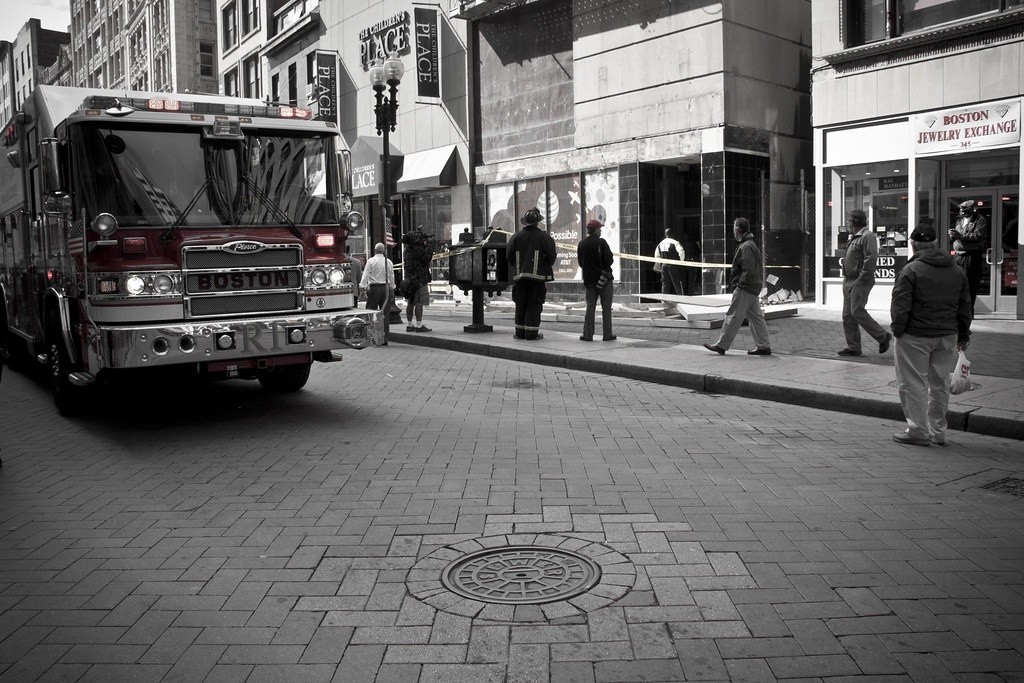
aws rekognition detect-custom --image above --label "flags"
[386,218,394,246]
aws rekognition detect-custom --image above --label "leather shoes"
[704,342,725,355]
[747,347,771,355]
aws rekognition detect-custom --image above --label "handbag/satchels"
[949,346,974,395]
[398,276,420,299]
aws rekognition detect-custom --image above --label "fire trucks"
[0,84,390,425]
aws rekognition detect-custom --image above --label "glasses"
[846,219,853,224]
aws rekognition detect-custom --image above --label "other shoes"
[525,333,544,340]
[879,332,892,353]
[414,325,432,332]
[893,431,930,446]
[603,335,616,341]
[513,334,525,339]
[406,325,415,332]
[580,335,593,341]
[838,348,862,356]
[930,434,945,445]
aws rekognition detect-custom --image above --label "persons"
[577,220,617,342]
[654,228,685,303]
[836,209,892,356]
[506,207,557,340]
[346,242,396,312]
[948,200,986,303]
[403,236,434,332]
[703,219,771,356]
[890,222,973,446]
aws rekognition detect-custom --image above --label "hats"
[957,199,975,214]
[520,206,544,225]
[587,220,605,229]
[908,222,937,243]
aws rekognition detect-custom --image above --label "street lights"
[363,49,403,324]
